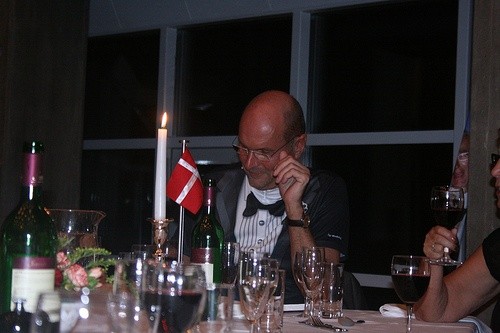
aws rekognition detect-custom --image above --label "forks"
[309,316,348,333]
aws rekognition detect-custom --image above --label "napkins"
[379,303,492,333]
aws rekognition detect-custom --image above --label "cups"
[320,262,344,319]
[260,268,285,333]
[107,249,207,333]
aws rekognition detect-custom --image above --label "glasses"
[231,135,300,162]
[491,153,500,169]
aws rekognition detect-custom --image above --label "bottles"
[0,139,60,333]
[189,177,225,322]
[35,207,152,332]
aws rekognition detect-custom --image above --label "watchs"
[285,214,311,228]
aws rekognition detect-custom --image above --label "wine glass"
[219,242,326,333]
[428,185,466,267]
[389,255,432,332]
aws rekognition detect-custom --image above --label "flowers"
[57,235,136,293]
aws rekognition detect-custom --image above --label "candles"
[154,112,168,220]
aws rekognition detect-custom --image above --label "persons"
[412,126,500,333]
[160,90,356,304]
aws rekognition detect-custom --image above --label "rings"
[432,241,436,250]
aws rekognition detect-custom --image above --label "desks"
[71,284,478,333]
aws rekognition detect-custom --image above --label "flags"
[166,146,204,213]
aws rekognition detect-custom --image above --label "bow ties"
[242,191,285,217]
